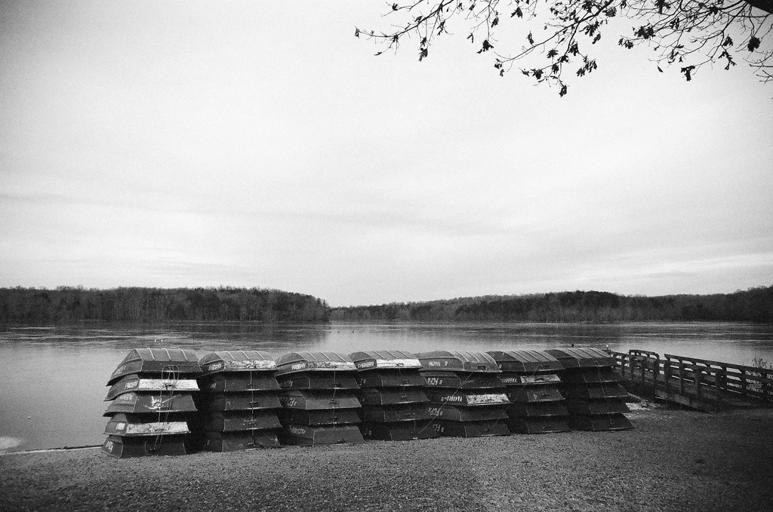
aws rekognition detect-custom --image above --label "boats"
[280,396,364,412]
[498,375,564,385]
[554,367,627,385]
[200,393,283,412]
[483,350,566,373]
[544,347,620,368]
[506,390,569,404]
[423,390,514,408]
[556,384,632,400]
[427,375,509,391]
[348,351,424,371]
[354,388,432,407]
[545,351,619,370]
[419,372,507,390]
[360,374,429,390]
[499,387,567,404]
[561,370,627,387]
[569,402,633,416]
[502,402,573,418]
[275,372,361,391]
[430,391,516,408]
[509,417,572,433]
[277,374,363,393]
[495,373,565,387]
[363,422,440,443]
[185,411,284,433]
[190,392,283,412]
[349,370,428,388]
[364,407,438,422]
[348,352,424,375]
[572,414,634,432]
[355,421,448,441]
[564,414,635,432]
[280,411,364,429]
[505,419,572,435]
[191,430,282,449]
[183,433,286,452]
[425,405,511,422]
[437,406,511,421]
[569,391,631,400]
[489,352,563,372]
[273,390,363,411]
[200,412,283,435]
[365,390,432,406]
[273,410,363,427]
[562,401,633,417]
[356,405,444,423]
[509,404,570,418]
